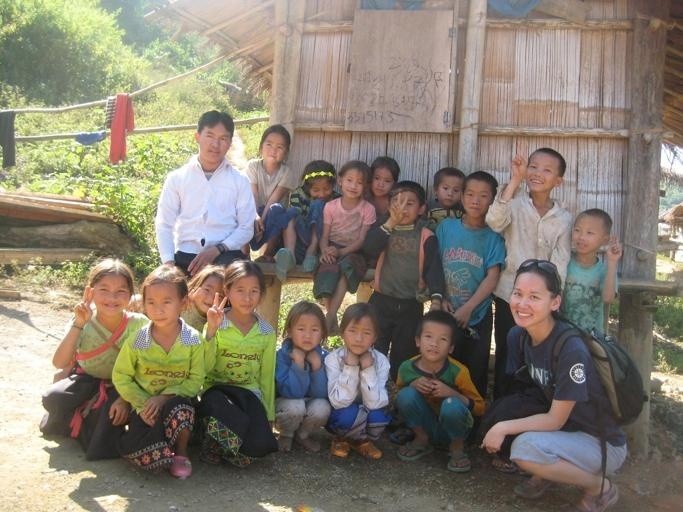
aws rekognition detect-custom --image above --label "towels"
[104,95,117,128]
[109,92,135,164]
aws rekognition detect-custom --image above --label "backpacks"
[550,317,648,425]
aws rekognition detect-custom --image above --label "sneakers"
[561,476,618,512]
[170,455,192,477]
[515,478,550,500]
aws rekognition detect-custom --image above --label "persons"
[396,310,484,473]
[480,258,627,511]
[110,263,204,480]
[484,139,575,403]
[361,156,399,282]
[275,160,341,282]
[556,209,622,342]
[313,160,375,335]
[424,168,467,233]
[195,260,277,467]
[134,265,227,331]
[245,125,292,261]
[323,302,390,459]
[432,171,505,402]
[39,258,150,461]
[365,181,446,382]
[273,301,328,453]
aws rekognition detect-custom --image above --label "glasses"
[518,258,557,275]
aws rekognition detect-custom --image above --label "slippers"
[397,441,435,461]
[447,452,471,472]
[276,248,318,273]
[332,440,383,459]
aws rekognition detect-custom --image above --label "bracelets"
[215,244,224,254]
[429,302,440,306]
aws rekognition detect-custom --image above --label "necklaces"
[155,110,256,280]
